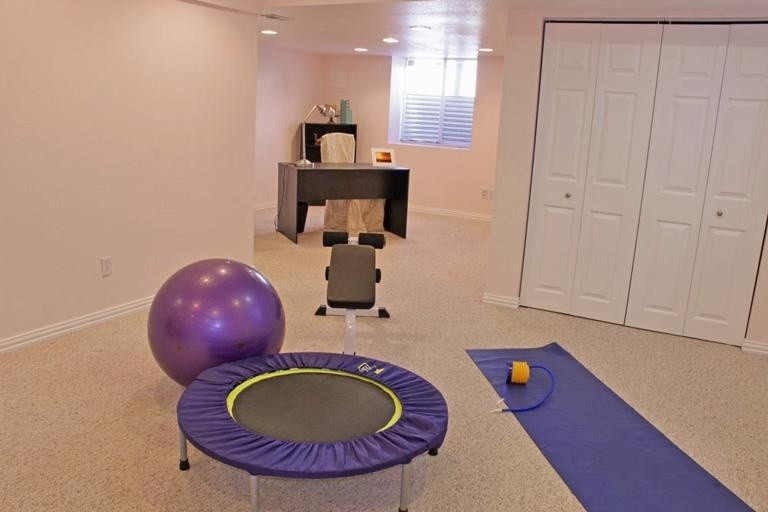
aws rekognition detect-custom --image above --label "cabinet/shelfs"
[299,120,358,164]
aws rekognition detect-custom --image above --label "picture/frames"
[371,147,396,167]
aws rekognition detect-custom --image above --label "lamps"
[298,103,335,167]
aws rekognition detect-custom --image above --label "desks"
[276,162,411,245]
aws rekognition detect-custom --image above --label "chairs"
[319,133,384,232]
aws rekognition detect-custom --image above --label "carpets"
[466,341,752,512]
